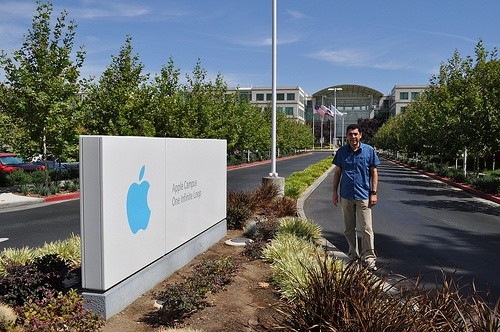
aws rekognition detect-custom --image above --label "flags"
[331,105,343,117]
[321,105,334,118]
[314,105,325,116]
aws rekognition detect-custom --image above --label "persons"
[333,124,380,271]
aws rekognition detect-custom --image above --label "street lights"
[341,112,348,146]
[327,87,343,155]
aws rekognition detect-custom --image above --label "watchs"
[371,191,378,195]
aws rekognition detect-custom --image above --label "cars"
[36,153,79,182]
[0,152,45,188]
